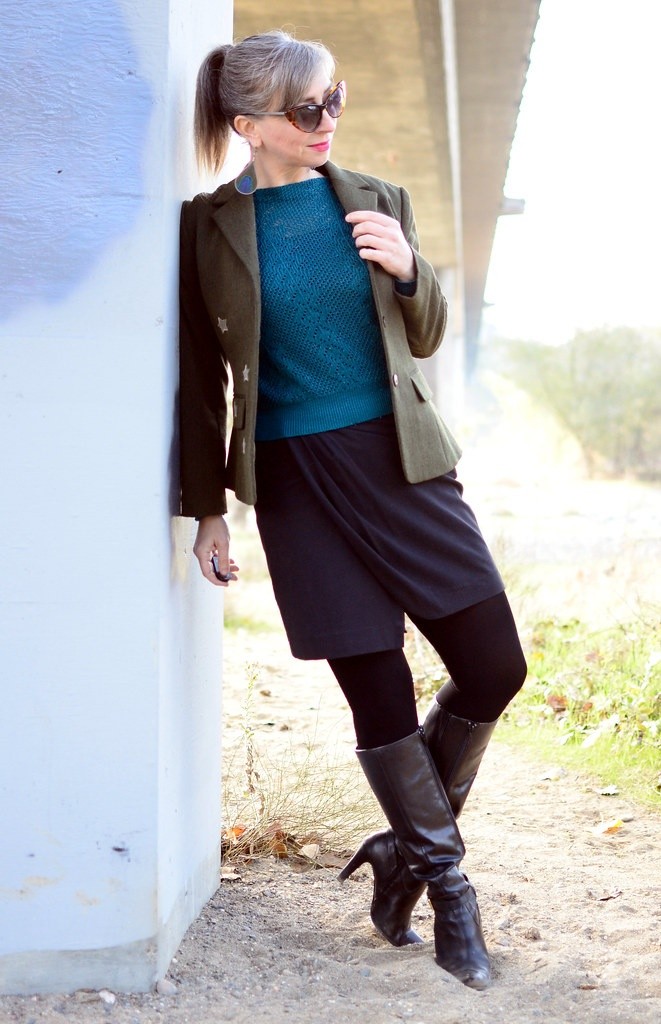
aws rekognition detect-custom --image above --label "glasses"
[237,79,346,133]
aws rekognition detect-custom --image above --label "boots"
[337,692,500,948]
[354,726,491,990]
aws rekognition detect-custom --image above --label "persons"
[177,30,528,989]
